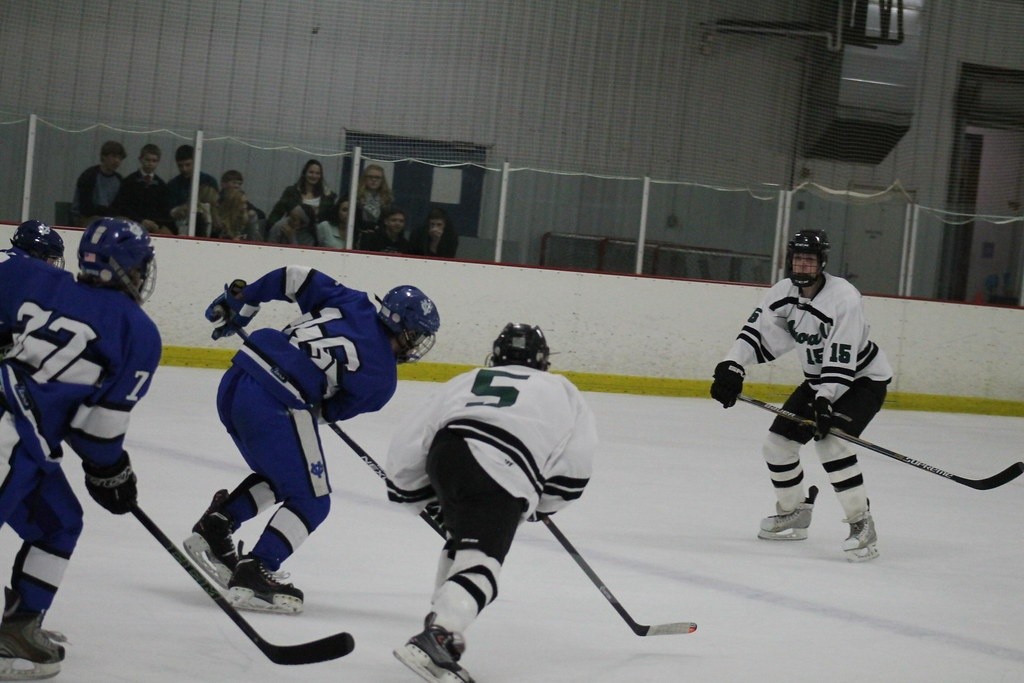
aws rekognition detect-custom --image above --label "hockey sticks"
[213,305,448,541]
[712,374,1024,491]
[64,435,355,665]
[542,516,697,637]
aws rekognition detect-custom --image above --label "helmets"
[492,323,551,372]
[77,217,158,306]
[785,230,831,287]
[10,220,65,270]
[376,284,440,365]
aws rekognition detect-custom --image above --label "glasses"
[364,175,382,179]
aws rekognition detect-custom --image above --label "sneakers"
[757,486,818,541]
[182,514,238,590]
[224,561,303,615]
[840,497,879,563]
[0,586,65,680]
[392,612,475,683]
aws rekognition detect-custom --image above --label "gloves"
[82,450,138,515]
[205,279,260,340]
[710,361,747,410]
[807,396,833,442]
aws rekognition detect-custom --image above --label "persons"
[183,265,439,614]
[71,139,458,259]
[709,229,894,563]
[0,216,161,683]
[384,323,598,683]
